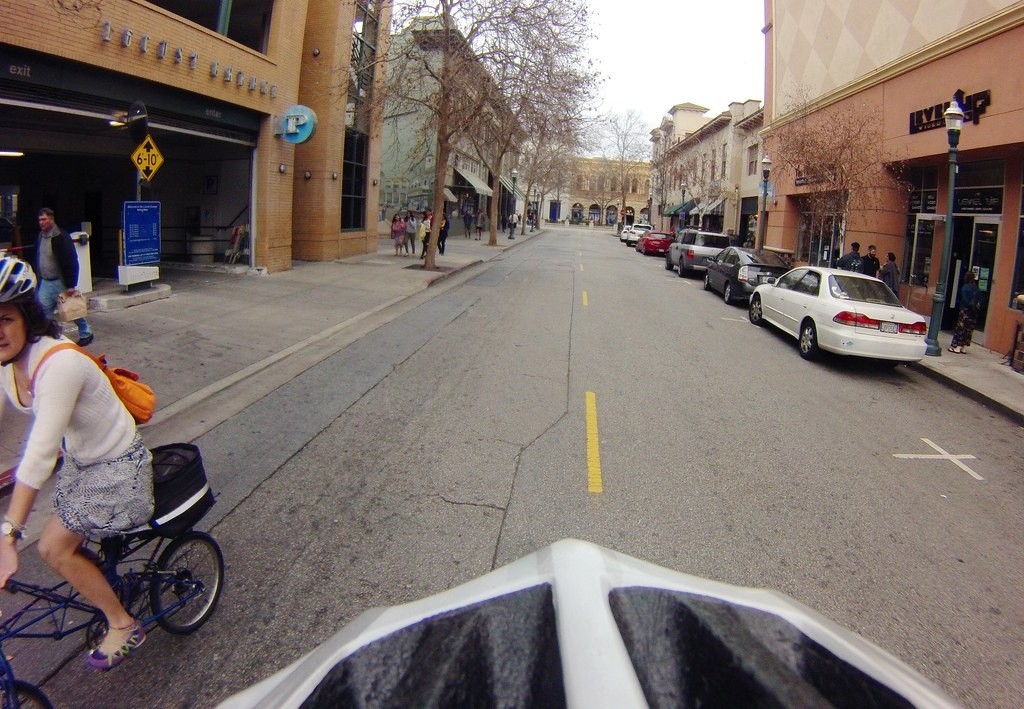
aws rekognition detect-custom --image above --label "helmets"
[0,249,38,304]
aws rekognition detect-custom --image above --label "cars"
[627,224,654,247]
[749,265,928,372]
[636,230,675,256]
[620,226,632,243]
[703,246,792,306]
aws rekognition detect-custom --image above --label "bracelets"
[4,515,26,531]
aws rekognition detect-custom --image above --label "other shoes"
[403,253,409,257]
[76,334,93,347]
[88,618,143,670]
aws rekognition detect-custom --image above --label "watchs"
[0,521,24,539]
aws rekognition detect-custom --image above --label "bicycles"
[0,442,225,709]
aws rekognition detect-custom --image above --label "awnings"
[498,175,526,200]
[454,166,493,197]
[662,197,724,217]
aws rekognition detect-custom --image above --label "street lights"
[507,168,519,239]
[925,101,963,356]
[679,183,688,229]
[759,155,773,250]
[529,184,537,232]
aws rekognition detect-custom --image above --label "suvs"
[665,226,731,279]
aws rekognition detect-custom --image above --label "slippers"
[960,349,966,354]
[948,348,960,354]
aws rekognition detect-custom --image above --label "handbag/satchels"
[391,230,400,239]
[145,443,217,536]
[58,289,88,323]
[30,343,156,425]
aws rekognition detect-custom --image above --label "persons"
[463,210,473,239]
[475,209,484,241]
[948,271,982,354]
[390,209,450,259]
[501,215,508,233]
[32,208,94,347]
[837,242,898,301]
[509,213,518,235]
[0,256,156,671]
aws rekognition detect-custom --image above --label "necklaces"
[14,361,34,398]
